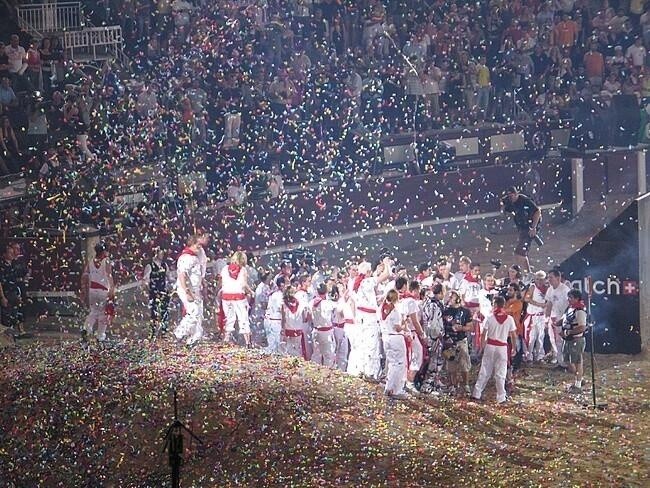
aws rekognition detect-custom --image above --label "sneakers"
[361,374,472,400]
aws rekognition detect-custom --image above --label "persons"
[143,229,587,403]
[0,0,650,229]
[504,187,541,274]
[79,242,116,343]
[0,242,30,339]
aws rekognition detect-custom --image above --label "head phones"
[516,264,521,278]
[456,291,461,303]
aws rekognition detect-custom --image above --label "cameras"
[491,258,502,269]
